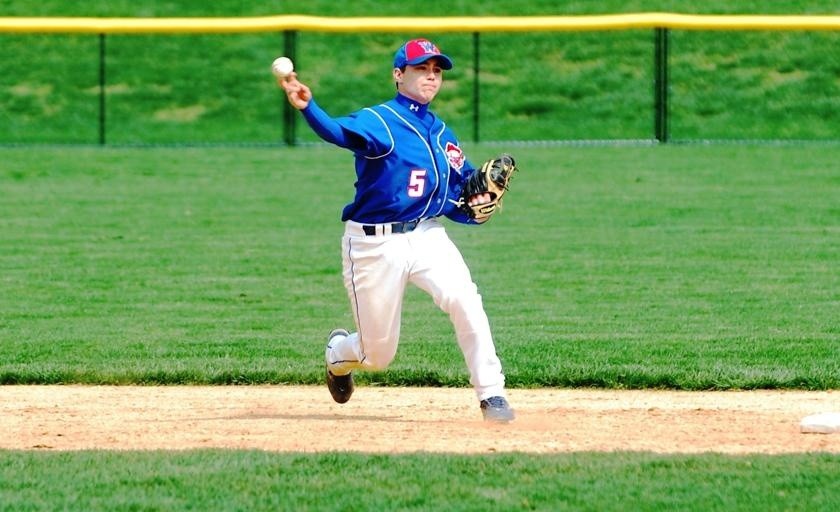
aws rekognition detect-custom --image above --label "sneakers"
[480,397,510,424]
[326,329,354,403]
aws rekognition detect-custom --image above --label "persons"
[278,38,516,423]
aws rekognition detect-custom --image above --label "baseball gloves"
[463,155,516,218]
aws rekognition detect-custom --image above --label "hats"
[394,39,453,70]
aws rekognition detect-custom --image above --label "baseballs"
[272,57,294,79]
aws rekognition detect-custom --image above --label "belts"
[363,215,436,235]
